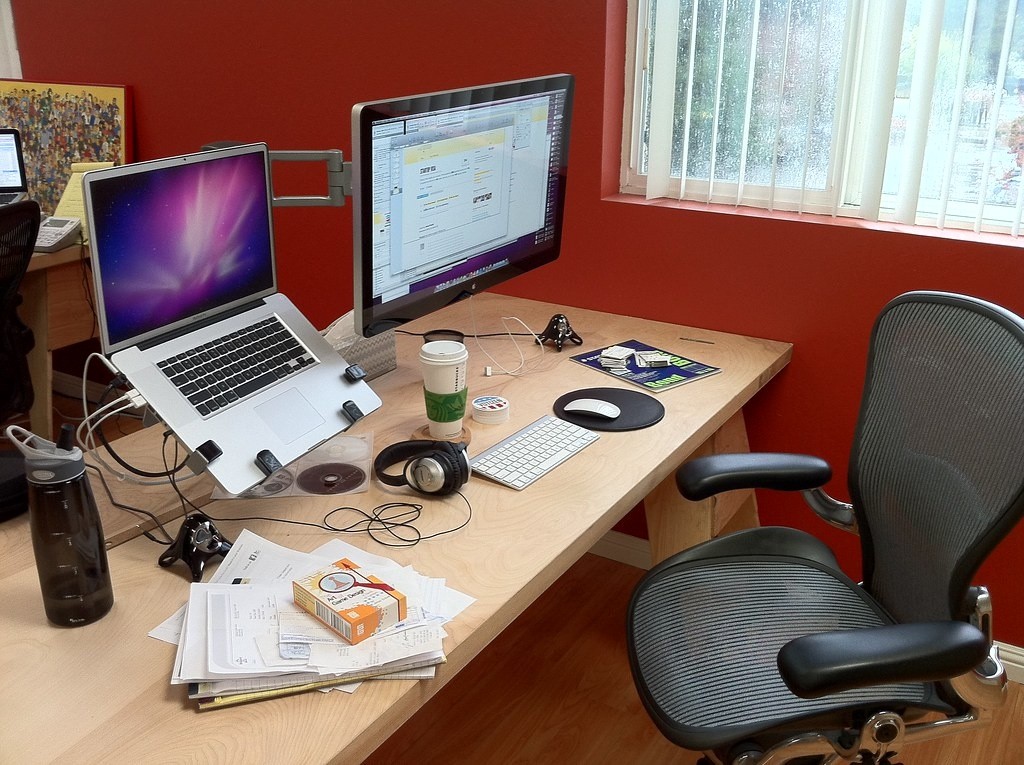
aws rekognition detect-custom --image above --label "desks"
[1,293,794,764]
[16,243,101,443]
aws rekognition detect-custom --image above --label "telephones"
[31,216,83,253]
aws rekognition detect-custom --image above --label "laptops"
[1,128,28,208]
[82,142,383,498]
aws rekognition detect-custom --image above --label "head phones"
[374,439,472,496]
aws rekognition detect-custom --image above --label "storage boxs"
[293,559,408,647]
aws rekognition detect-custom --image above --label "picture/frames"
[0,78,134,221]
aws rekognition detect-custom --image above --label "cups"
[417,340,469,440]
[424,329,467,346]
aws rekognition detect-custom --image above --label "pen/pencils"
[680,337,714,347]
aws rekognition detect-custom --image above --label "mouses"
[564,399,621,420]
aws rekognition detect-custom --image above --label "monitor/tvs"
[351,72,576,340]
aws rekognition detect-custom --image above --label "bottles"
[6,422,113,627]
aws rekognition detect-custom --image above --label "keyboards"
[469,414,600,491]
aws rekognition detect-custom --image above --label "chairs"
[625,290,1024,765]
[0,200,41,418]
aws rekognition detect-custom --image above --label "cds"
[235,469,294,498]
[297,462,366,495]
